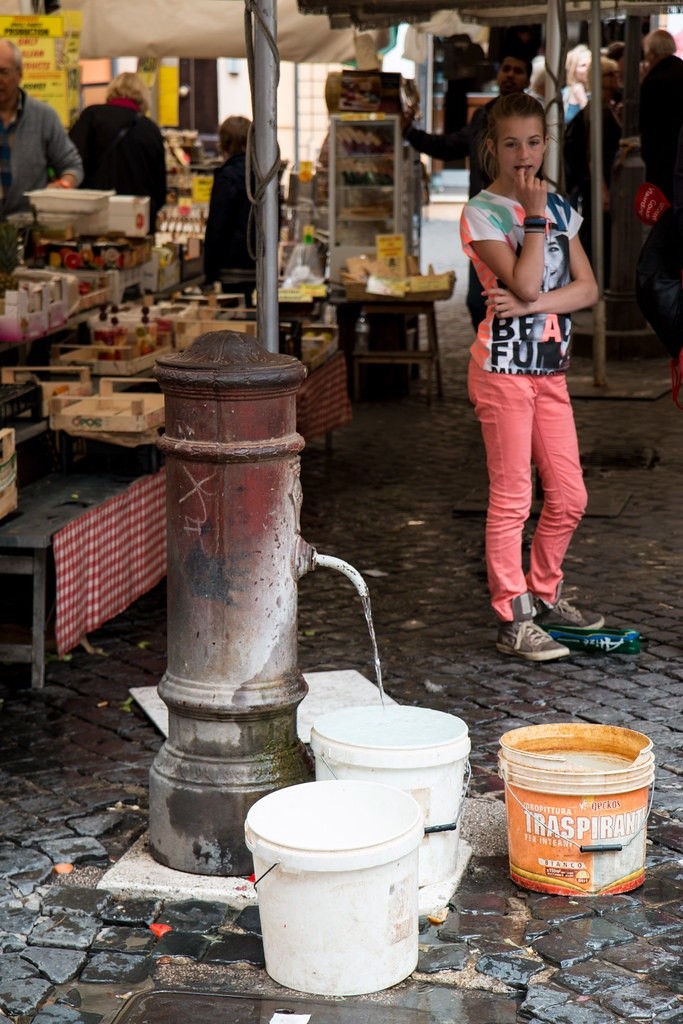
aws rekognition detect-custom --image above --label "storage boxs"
[0,10,336,523]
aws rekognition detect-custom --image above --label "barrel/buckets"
[245,781,419,997]
[310,706,473,886]
[498,723,656,897]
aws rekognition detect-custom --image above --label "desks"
[0,349,352,690]
[330,295,443,411]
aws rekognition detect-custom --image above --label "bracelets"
[524,217,546,227]
[55,178,72,188]
[525,227,545,233]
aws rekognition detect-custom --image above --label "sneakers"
[496,592,570,662]
[532,579,605,631]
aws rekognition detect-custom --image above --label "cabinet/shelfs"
[328,115,403,284]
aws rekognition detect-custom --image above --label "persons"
[68,73,168,238]
[0,39,84,258]
[460,93,605,662]
[402,30,683,351]
[201,118,262,309]
